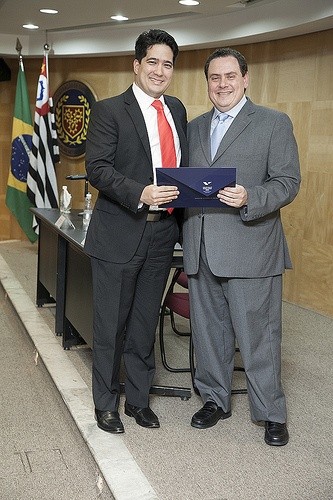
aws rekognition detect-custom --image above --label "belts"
[146,210,171,222]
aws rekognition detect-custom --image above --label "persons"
[85,30,188,434]
[182,48,301,446]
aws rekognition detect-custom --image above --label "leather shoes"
[94,407,125,433]
[124,400,161,428]
[264,421,289,446]
[191,401,232,429]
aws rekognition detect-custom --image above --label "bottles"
[83,191,94,214]
[60,185,71,213]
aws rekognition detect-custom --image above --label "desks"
[30,207,191,400]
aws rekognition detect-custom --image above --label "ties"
[152,100,178,214]
[210,113,229,161]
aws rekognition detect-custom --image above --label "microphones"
[66,174,90,181]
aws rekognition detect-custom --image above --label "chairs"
[160,256,247,395]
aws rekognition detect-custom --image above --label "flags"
[5,55,39,245]
[27,51,61,235]
